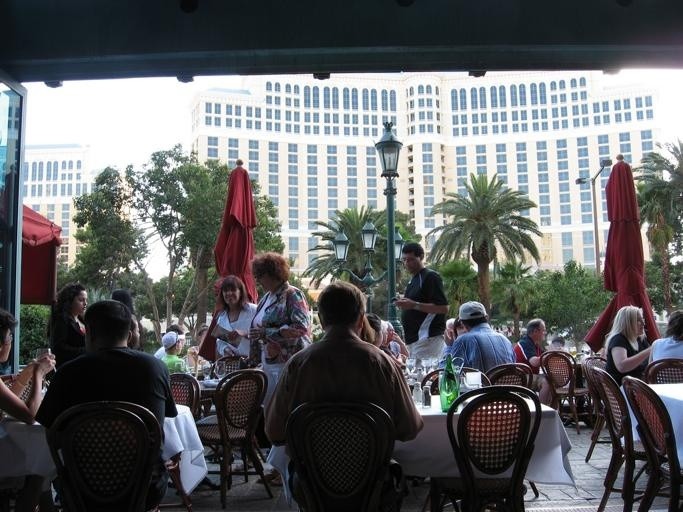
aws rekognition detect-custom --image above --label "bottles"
[439,353,458,413]
[411,382,422,404]
[421,384,431,407]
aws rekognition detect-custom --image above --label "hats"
[458,300,488,320]
[162,331,186,350]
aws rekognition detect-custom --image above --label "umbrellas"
[584,154,658,354]
[215,159,259,303]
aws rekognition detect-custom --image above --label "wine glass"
[404,356,416,379]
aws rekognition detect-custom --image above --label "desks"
[618,383,682,460]
[4,404,207,498]
[392,395,580,491]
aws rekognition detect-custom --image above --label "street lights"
[368,116,405,341]
[328,217,405,314]
[575,156,613,277]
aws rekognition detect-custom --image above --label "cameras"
[36,349,51,359]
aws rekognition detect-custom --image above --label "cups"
[420,357,432,374]
[173,359,226,384]
[35,346,51,360]
[568,347,576,356]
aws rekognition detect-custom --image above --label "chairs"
[622,376,680,511]
[48,401,167,510]
[163,320,608,433]
[646,358,680,384]
[447,385,541,512]
[198,369,275,510]
[280,402,402,512]
[590,366,667,512]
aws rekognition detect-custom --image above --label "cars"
[184,330,192,340]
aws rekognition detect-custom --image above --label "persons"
[453,317,466,336]
[440,301,516,374]
[1,253,424,512]
[396,243,450,346]
[514,319,553,405]
[604,305,651,387]
[648,310,683,384]
